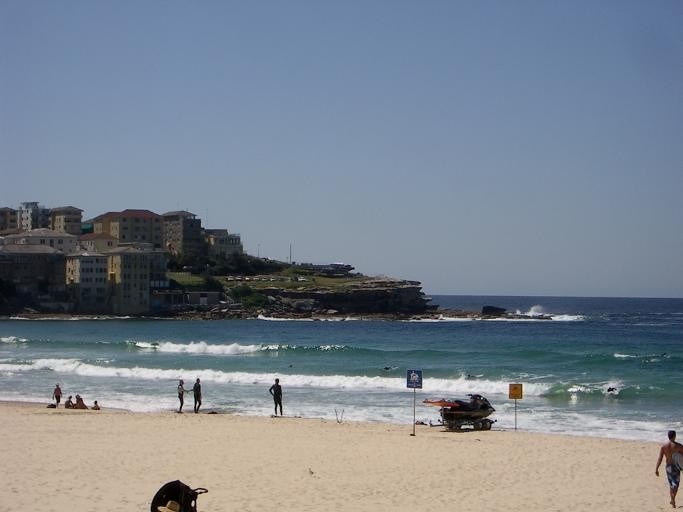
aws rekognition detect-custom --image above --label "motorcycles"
[438,392,498,432]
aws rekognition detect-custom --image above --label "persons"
[176,377,189,413]
[64,395,73,409]
[268,377,282,416]
[51,383,62,408]
[76,398,87,408]
[90,399,101,409]
[656,428,683,508]
[73,394,81,409]
[192,377,202,414]
